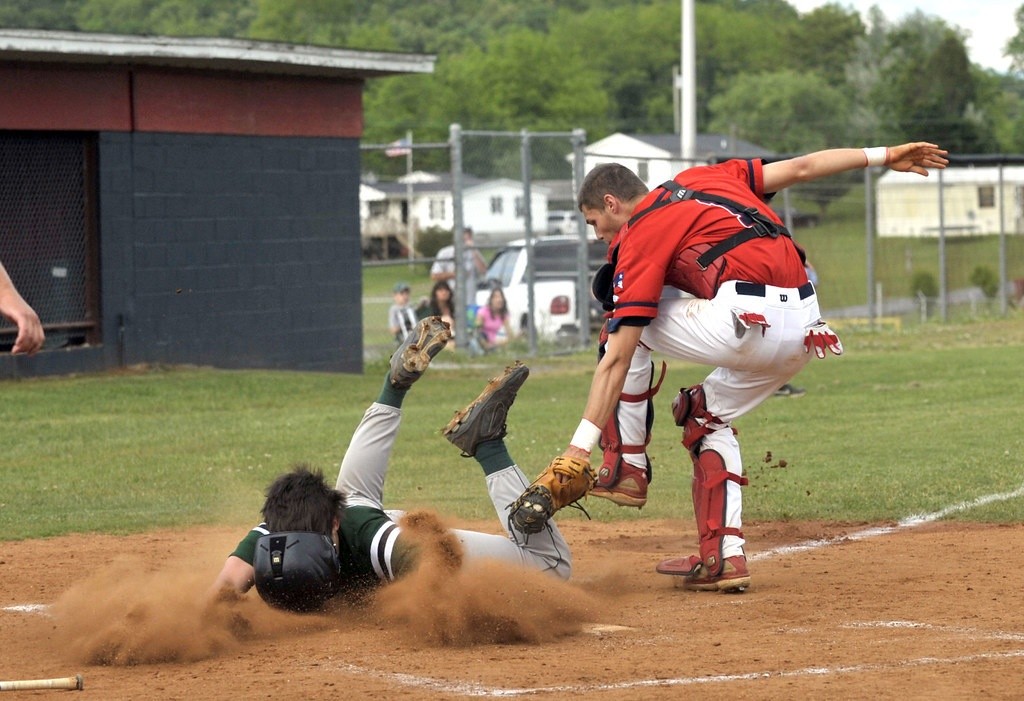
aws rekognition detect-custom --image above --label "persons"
[0,261,45,356]
[552,142,950,594]
[207,316,572,616]
[390,227,514,355]
[774,259,817,398]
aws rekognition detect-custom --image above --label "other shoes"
[774,385,804,401]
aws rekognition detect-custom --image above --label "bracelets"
[571,418,602,454]
[862,146,888,168]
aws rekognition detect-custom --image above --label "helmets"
[253,531,343,615]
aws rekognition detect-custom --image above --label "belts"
[735,281,814,300]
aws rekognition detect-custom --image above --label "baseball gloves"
[508,454,598,535]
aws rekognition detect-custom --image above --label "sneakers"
[440,360,529,458]
[591,458,648,508]
[682,555,752,590]
[389,316,451,391]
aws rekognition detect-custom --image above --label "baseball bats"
[0,673,84,691]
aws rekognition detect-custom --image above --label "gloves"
[804,324,844,359]
[731,309,770,339]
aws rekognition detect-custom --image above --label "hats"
[393,284,410,293]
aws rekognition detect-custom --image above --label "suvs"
[474,236,610,344]
